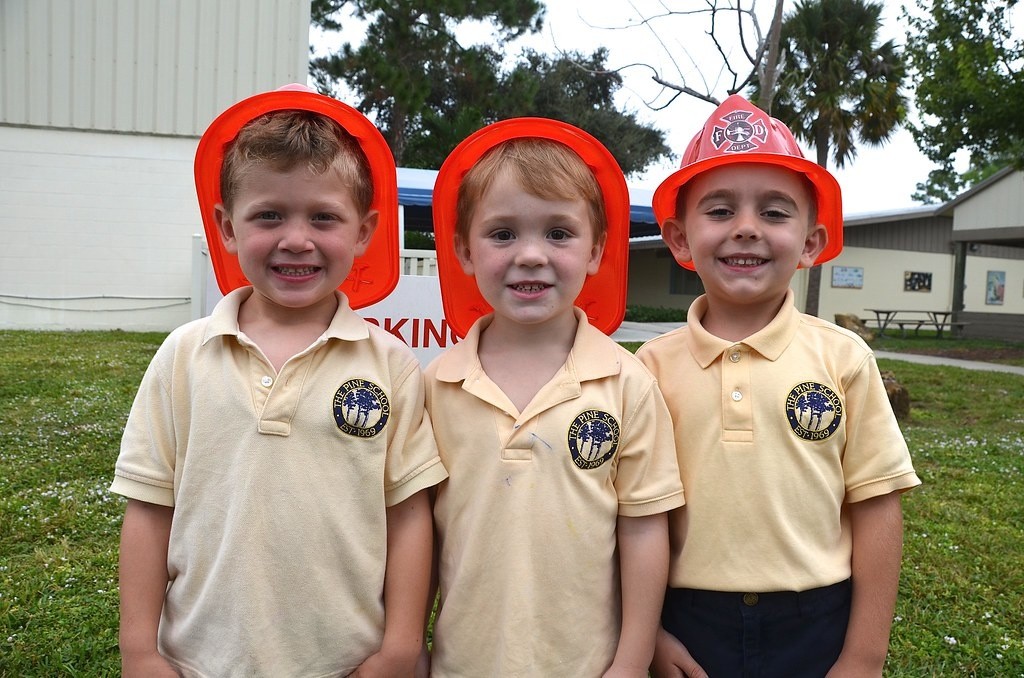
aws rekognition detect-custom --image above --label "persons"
[420,117,684,678]
[632,95,921,678]
[108,83,448,678]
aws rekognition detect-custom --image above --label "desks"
[863,308,956,341]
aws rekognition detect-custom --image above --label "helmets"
[432,118,630,339]
[194,85,399,310]
[651,95,844,271]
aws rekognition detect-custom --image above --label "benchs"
[860,319,933,332]
[891,321,973,342]
